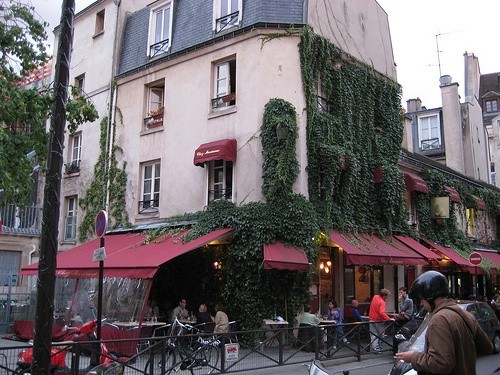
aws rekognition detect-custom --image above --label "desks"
[180,320,197,348]
[361,316,369,322]
[317,320,336,348]
[388,313,399,319]
[114,320,166,355]
[264,319,289,348]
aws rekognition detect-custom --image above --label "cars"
[392,299,500,355]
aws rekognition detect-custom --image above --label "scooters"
[14,318,124,375]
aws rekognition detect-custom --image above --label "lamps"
[320,261,331,273]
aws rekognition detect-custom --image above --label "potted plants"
[147,120,163,129]
[150,106,164,120]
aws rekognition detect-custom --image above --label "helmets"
[408,270,450,300]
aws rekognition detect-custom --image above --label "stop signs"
[469,252,483,266]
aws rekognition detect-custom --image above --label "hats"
[381,289,390,295]
[352,300,359,307]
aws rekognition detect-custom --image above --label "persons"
[299,304,321,328]
[368,289,395,352]
[447,288,500,317]
[169,297,191,333]
[325,300,350,349]
[210,302,230,334]
[395,271,493,375]
[350,300,366,331]
[396,287,413,320]
[196,304,214,333]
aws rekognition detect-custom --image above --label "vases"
[221,94,235,103]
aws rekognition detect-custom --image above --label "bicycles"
[143,317,220,375]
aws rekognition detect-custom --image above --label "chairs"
[136,324,171,354]
[189,320,241,353]
[296,323,320,352]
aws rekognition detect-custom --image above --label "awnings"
[442,185,460,203]
[21,221,240,281]
[470,194,485,211]
[392,233,443,262]
[259,239,309,272]
[399,171,429,194]
[420,237,496,270]
[193,139,237,166]
[326,227,427,268]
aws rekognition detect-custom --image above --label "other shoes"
[343,338,350,344]
[333,346,337,350]
[374,348,382,354]
